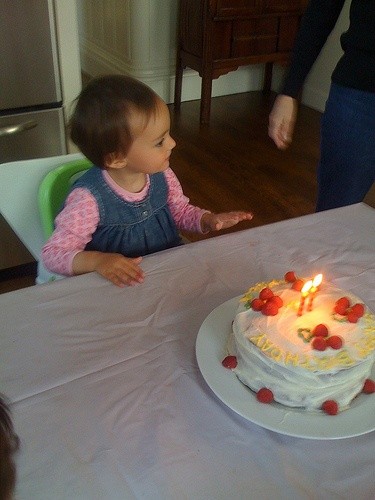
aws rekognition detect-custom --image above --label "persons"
[0,393,20,500]
[268,0,375,212]
[43,75,253,287]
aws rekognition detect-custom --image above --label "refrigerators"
[0,0,67,272]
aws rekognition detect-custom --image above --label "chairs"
[38,158,95,242]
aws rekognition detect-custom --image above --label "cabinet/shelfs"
[173,0,311,130]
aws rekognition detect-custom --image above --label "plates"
[194,293,375,440]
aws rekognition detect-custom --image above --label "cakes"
[221,272,375,415]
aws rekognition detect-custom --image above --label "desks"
[0,202,375,500]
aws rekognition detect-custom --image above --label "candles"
[305,273,324,311]
[296,279,312,317]
